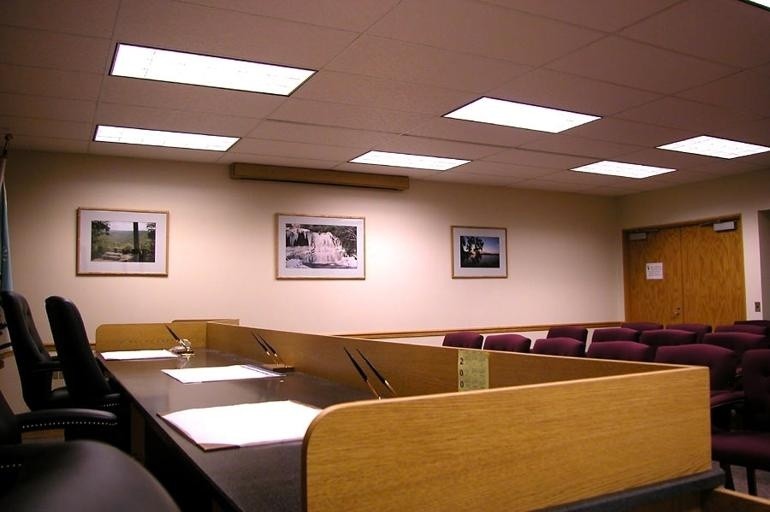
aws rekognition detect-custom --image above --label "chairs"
[442,319,769,496]
[1,289,182,511]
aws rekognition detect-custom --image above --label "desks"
[94,319,725,512]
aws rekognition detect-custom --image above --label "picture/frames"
[76,206,170,279]
[273,212,366,281]
[450,225,509,280]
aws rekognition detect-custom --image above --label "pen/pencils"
[164,324,187,348]
[343,347,384,399]
[253,334,288,367]
[357,349,399,397]
